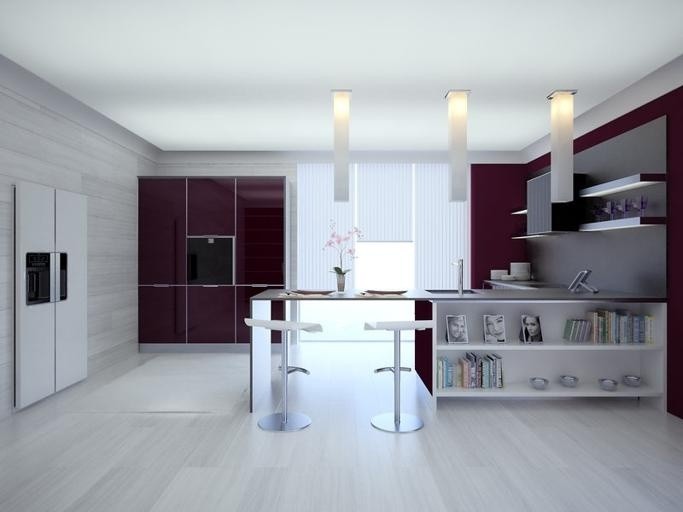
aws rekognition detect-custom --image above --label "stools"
[245,318,323,431]
[365,320,434,433]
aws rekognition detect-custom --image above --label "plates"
[490,261,531,282]
[366,289,407,296]
[291,289,336,296]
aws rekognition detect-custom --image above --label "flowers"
[323,223,364,274]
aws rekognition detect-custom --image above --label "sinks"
[528,284,570,289]
[426,290,475,294]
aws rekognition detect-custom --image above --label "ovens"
[187,236,235,286]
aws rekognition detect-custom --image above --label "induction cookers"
[511,282,569,289]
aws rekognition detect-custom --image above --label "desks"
[249,289,429,413]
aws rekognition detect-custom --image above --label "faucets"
[452,258,463,294]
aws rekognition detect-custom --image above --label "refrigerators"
[10,179,89,411]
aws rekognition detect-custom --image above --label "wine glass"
[593,194,647,221]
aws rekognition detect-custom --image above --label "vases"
[337,274,345,292]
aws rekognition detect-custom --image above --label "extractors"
[525,171,590,235]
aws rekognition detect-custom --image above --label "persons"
[448,317,466,342]
[486,316,505,342]
[524,316,543,343]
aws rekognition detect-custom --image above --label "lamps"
[332,90,352,203]
[547,89,577,203]
[445,90,471,202]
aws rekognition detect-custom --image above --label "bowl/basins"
[528,377,548,390]
[560,375,578,388]
[597,377,617,392]
[623,375,639,387]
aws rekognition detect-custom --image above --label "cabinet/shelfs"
[510,172,667,240]
[432,300,668,416]
[138,176,286,355]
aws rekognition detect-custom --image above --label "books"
[563,307,654,345]
[436,351,503,389]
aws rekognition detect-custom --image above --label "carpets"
[66,352,250,411]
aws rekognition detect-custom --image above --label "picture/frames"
[446,315,468,344]
[484,315,506,344]
[521,315,544,344]
